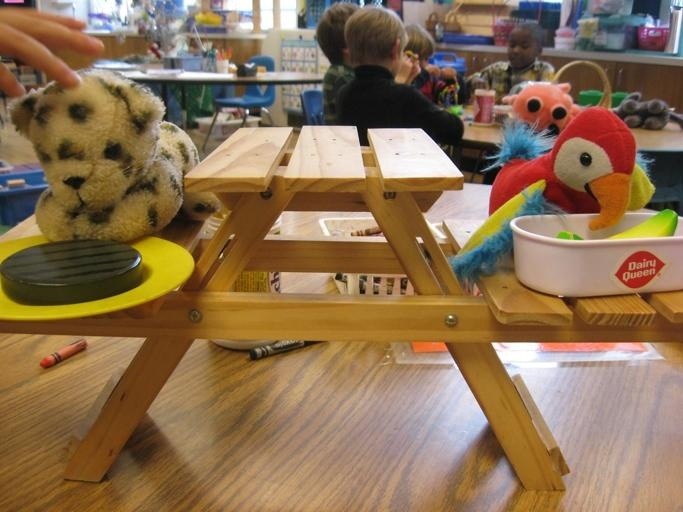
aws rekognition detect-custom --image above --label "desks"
[1,123,683,512]
[120,72,324,128]
[440,105,683,213]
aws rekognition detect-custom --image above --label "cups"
[473,89,494,125]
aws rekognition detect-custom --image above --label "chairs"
[203,56,275,153]
[300,87,326,125]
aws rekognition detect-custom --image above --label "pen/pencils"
[215,45,232,60]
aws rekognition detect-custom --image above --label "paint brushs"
[250,340,327,360]
[351,227,382,236]
[40,339,87,369]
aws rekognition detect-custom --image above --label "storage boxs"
[639,26,670,51]
[0,170,50,227]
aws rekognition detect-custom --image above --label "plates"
[0,235,195,320]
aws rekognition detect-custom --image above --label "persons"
[465,21,555,105]
[403,23,458,105]
[336,5,464,145]
[315,3,420,124]
[0,7,105,97]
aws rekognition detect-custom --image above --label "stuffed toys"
[6,69,219,241]
[502,82,582,153]
[450,108,655,277]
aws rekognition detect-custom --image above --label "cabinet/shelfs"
[48,35,262,114]
[437,48,683,112]
